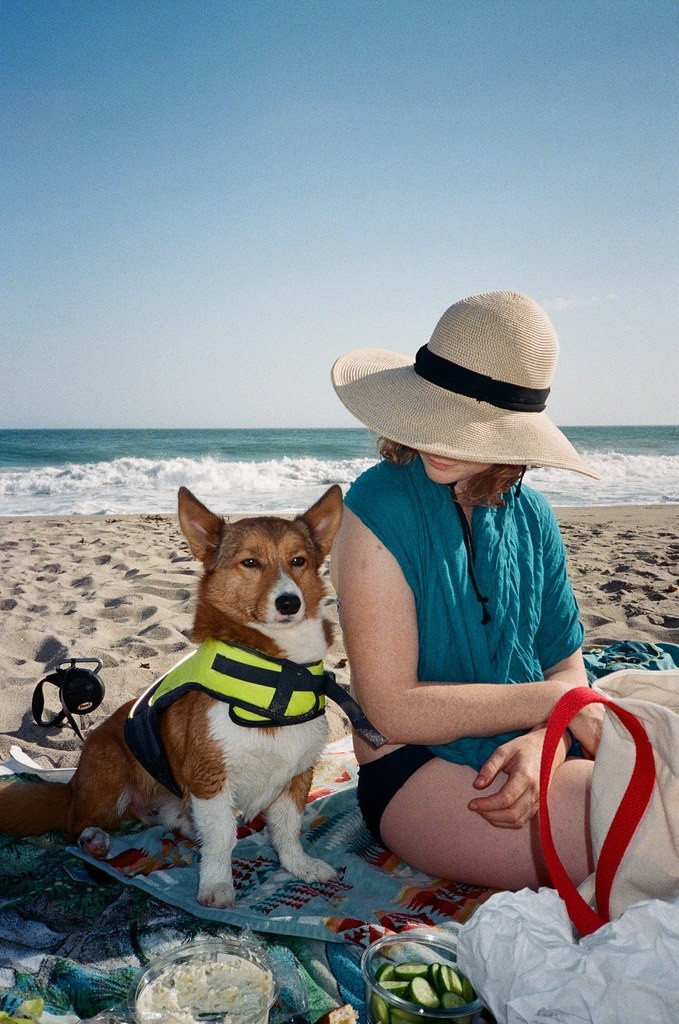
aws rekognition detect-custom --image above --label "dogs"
[0,483,345,910]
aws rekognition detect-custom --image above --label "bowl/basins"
[127,939,282,1024]
[361,934,483,1024]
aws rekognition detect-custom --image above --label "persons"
[331,292,603,889]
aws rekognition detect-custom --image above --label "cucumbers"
[369,961,480,1024]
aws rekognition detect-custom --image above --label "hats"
[331,290,603,481]
